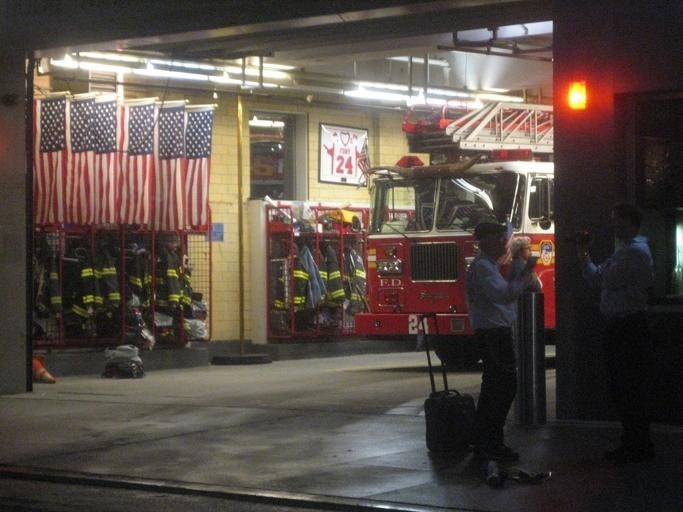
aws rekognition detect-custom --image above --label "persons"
[576,203,653,464]
[466,223,534,460]
[506,236,543,290]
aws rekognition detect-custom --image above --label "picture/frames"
[317,120,371,187]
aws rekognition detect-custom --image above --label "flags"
[33,92,218,234]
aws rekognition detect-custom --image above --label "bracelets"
[578,251,590,261]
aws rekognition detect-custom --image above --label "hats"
[473,222,508,239]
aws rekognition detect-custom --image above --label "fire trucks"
[353,97,556,365]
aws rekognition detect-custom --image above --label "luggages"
[420,310,475,452]
